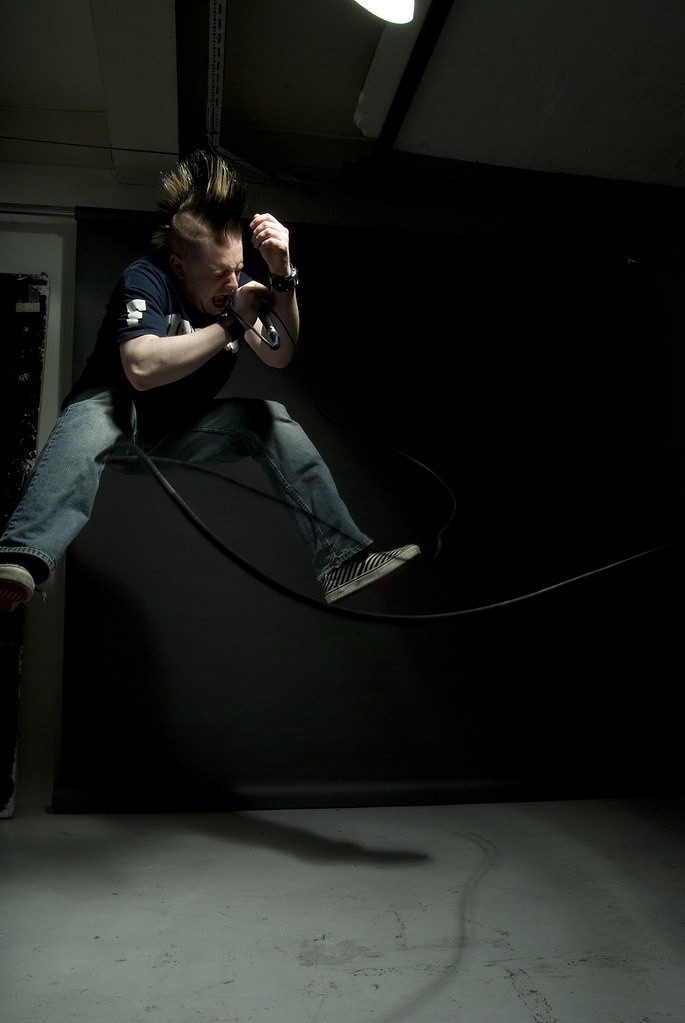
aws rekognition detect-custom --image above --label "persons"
[0,147,422,614]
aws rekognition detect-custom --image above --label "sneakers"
[0,563,35,613]
[320,543,422,606]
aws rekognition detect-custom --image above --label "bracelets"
[269,262,300,294]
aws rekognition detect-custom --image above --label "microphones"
[256,304,280,350]
